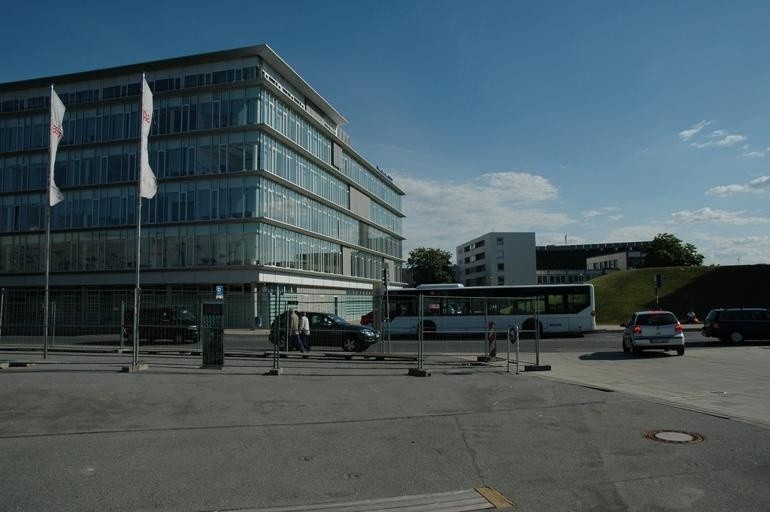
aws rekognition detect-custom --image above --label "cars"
[620,310,685,355]
[269,310,380,351]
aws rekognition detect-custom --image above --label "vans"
[702,308,770,345]
[124,309,200,345]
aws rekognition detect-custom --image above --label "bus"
[380,284,597,339]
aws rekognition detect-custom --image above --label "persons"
[296,312,311,351]
[283,310,306,359]
[687,309,696,324]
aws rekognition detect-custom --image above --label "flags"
[49,89,68,208]
[140,76,160,200]
